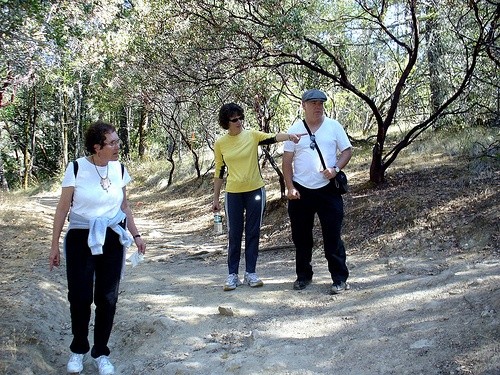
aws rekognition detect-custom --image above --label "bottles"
[214,209,222,235]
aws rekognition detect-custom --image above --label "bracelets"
[133,235,141,239]
[288,134,291,141]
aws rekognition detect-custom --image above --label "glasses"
[103,138,122,146]
[310,135,315,150]
[229,115,243,122]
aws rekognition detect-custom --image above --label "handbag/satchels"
[330,170,349,194]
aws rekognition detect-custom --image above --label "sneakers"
[93,356,114,375]
[66,352,88,373]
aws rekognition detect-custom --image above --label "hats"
[302,89,327,102]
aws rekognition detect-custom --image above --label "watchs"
[333,165,340,173]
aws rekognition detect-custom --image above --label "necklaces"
[92,156,111,193]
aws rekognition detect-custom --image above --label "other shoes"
[243,272,264,287]
[223,273,241,290]
[294,274,312,289]
[330,280,348,294]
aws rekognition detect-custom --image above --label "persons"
[282,90,352,294]
[49,121,147,375]
[212,103,308,291]
